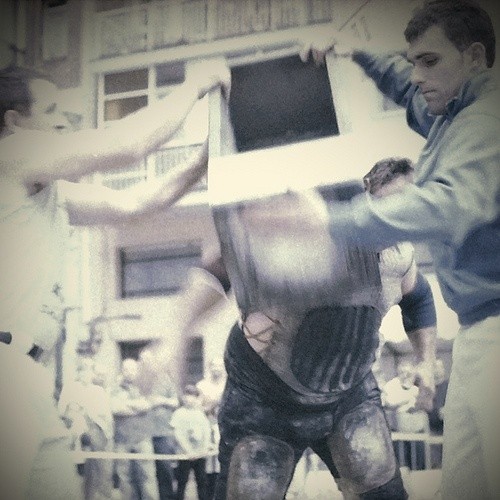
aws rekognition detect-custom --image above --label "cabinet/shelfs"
[206,41,389,321]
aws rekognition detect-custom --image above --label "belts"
[0,331,45,364]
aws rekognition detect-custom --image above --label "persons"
[144,149,442,500]
[245,3,500,500]
[56,348,446,500]
[2,63,232,500]
